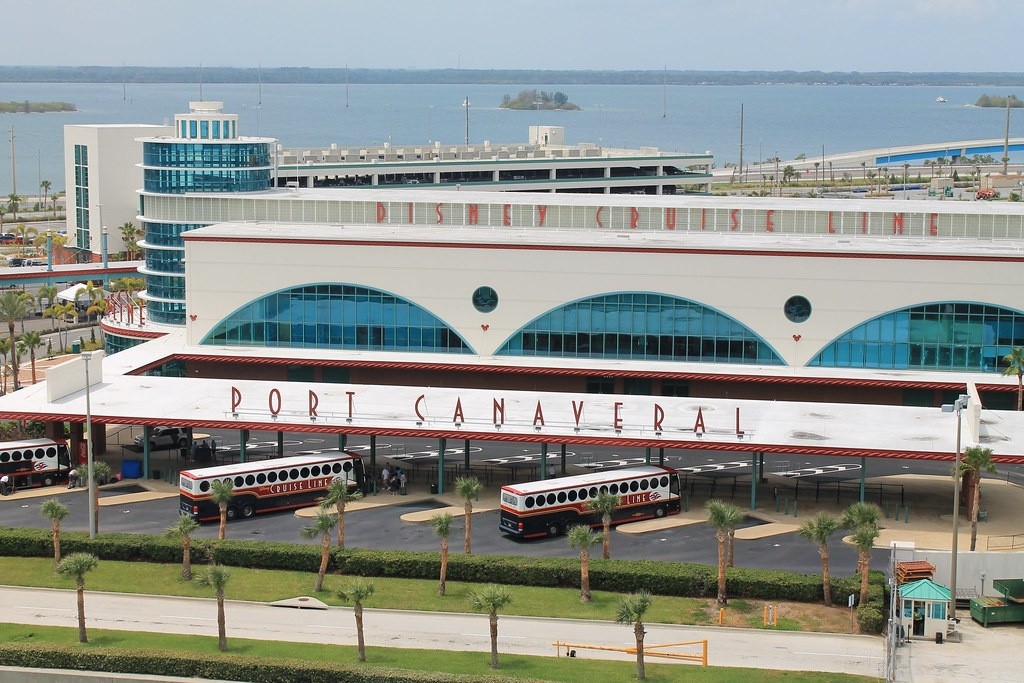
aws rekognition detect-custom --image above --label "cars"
[9,258,47,265]
[56,230,67,237]
[0,233,33,245]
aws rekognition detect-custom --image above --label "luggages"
[400,479,406,495]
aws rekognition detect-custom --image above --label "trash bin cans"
[121,459,143,479]
[152,470,160,479]
[431,484,438,494]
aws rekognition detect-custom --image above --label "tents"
[56,283,111,317]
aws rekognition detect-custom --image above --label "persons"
[367,462,407,496]
[548,463,556,479]
[67,469,77,489]
[0,473,10,496]
[189,439,216,463]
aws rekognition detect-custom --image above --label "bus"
[178,449,367,521]
[0,438,75,490]
[499,463,685,539]
[67,280,103,287]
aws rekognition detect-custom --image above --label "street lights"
[82,350,95,539]
[940,394,972,617]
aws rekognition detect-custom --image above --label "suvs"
[134,426,192,451]
